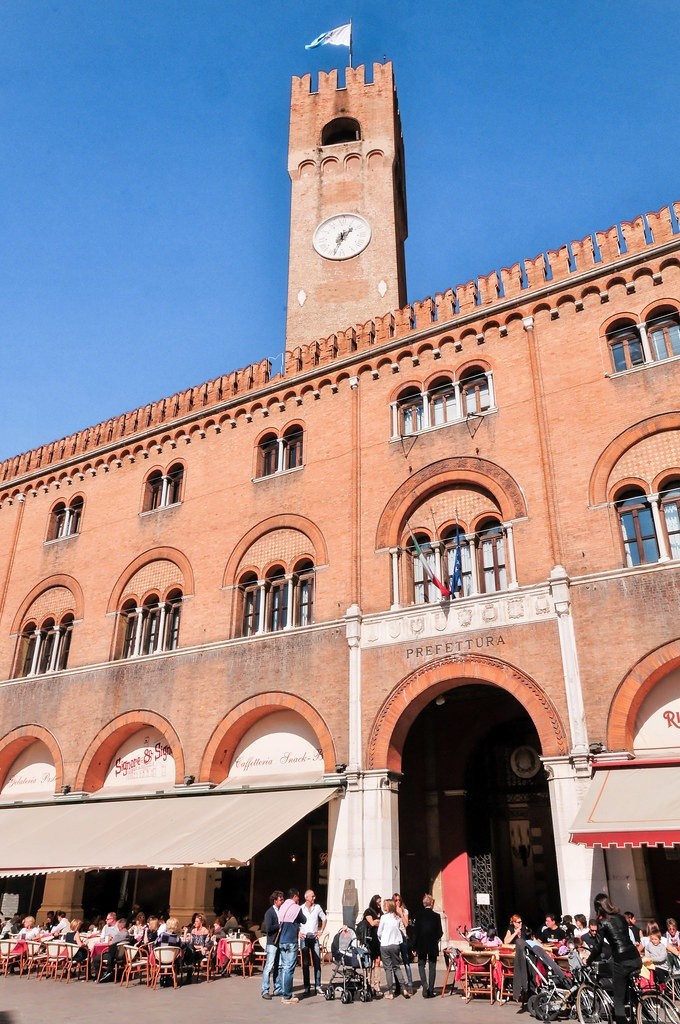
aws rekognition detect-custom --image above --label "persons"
[0,891,680,1024]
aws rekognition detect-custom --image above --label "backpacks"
[357,910,372,947]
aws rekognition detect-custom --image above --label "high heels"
[373,981,383,997]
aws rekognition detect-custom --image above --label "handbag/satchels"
[406,924,417,957]
[273,927,282,947]
[160,975,173,988]
[99,972,112,983]
[401,937,410,948]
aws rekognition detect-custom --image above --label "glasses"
[517,920,522,923]
[590,928,597,932]
[196,913,202,922]
[395,899,400,902]
[139,916,145,919]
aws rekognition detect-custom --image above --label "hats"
[158,915,166,923]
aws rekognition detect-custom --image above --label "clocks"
[310,214,372,261]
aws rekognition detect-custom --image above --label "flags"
[450,515,463,600]
[409,530,451,595]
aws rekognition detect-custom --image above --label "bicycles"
[531,963,680,1024]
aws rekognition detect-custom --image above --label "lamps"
[335,764,347,773]
[184,776,195,786]
[60,785,71,795]
[590,742,603,753]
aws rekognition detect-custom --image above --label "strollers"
[325,926,372,1004]
[521,947,590,1020]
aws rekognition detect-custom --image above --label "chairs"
[0,926,329,991]
[442,943,571,1006]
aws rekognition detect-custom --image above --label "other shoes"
[407,986,414,995]
[262,988,325,1004]
[423,984,434,998]
[404,991,410,999]
[385,994,394,1000]
[658,984,667,989]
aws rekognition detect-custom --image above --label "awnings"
[0,786,346,878]
[567,762,680,849]
